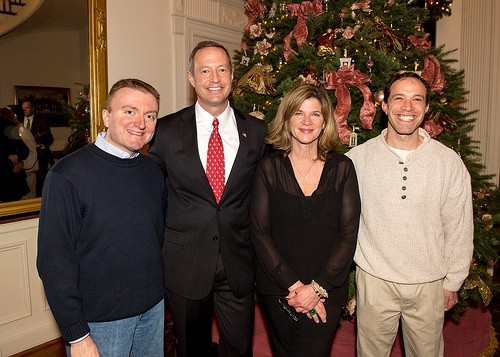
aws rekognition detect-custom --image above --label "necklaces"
[290,151,315,180]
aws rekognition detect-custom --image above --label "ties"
[26,119,30,129]
[205,117,225,205]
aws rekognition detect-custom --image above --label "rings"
[309,309,317,315]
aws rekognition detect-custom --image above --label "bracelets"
[310,280,328,299]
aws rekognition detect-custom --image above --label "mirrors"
[0,0,108,224]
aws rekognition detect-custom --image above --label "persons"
[248,83,362,357]
[0,98,55,207]
[149,41,269,357]
[342,72,475,357]
[34,79,169,357]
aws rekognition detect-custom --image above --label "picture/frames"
[13,84,71,127]
